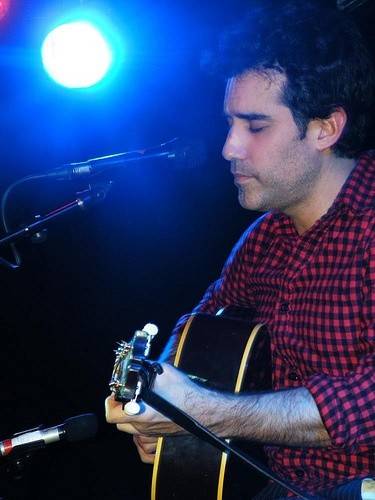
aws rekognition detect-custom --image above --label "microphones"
[0,414,98,456]
[47,137,195,182]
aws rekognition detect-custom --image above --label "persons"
[105,5,375,500]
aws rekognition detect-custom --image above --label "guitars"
[108,304,270,500]
